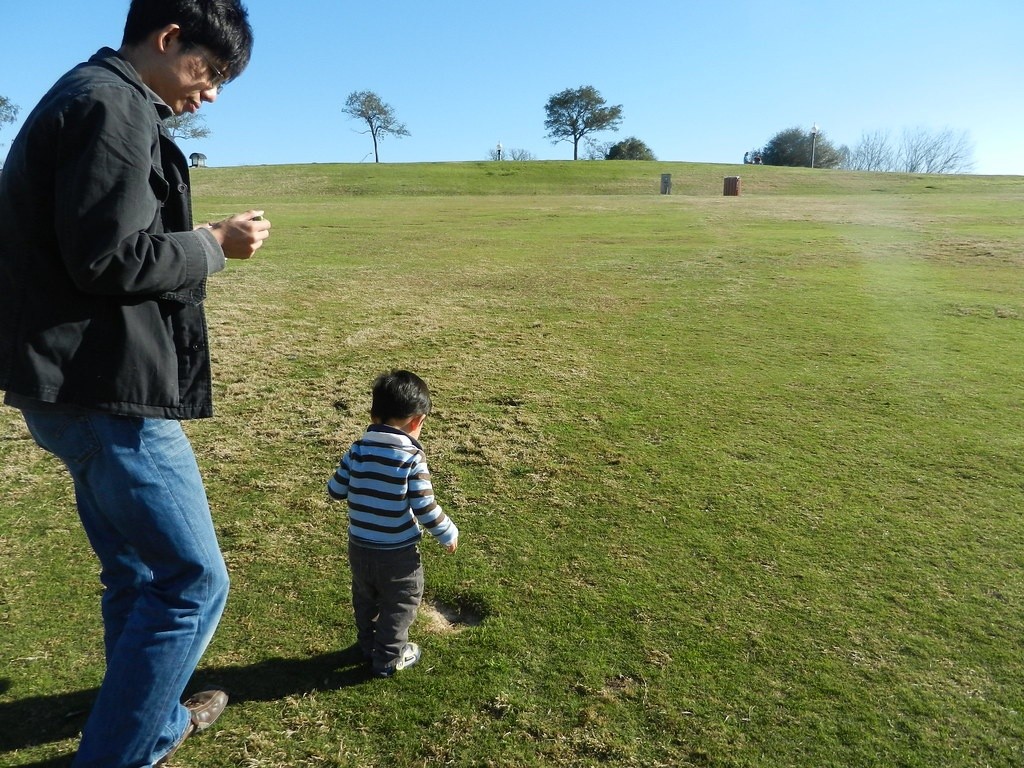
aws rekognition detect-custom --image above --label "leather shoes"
[160,690,229,762]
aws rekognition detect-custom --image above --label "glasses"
[191,41,226,95]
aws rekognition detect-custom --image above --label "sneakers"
[357,641,422,678]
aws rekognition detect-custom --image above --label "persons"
[327,370,460,678]
[0,0,272,767]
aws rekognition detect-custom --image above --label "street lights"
[810,121,817,168]
[496,144,501,160]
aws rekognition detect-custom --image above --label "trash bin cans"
[723,176,741,197]
